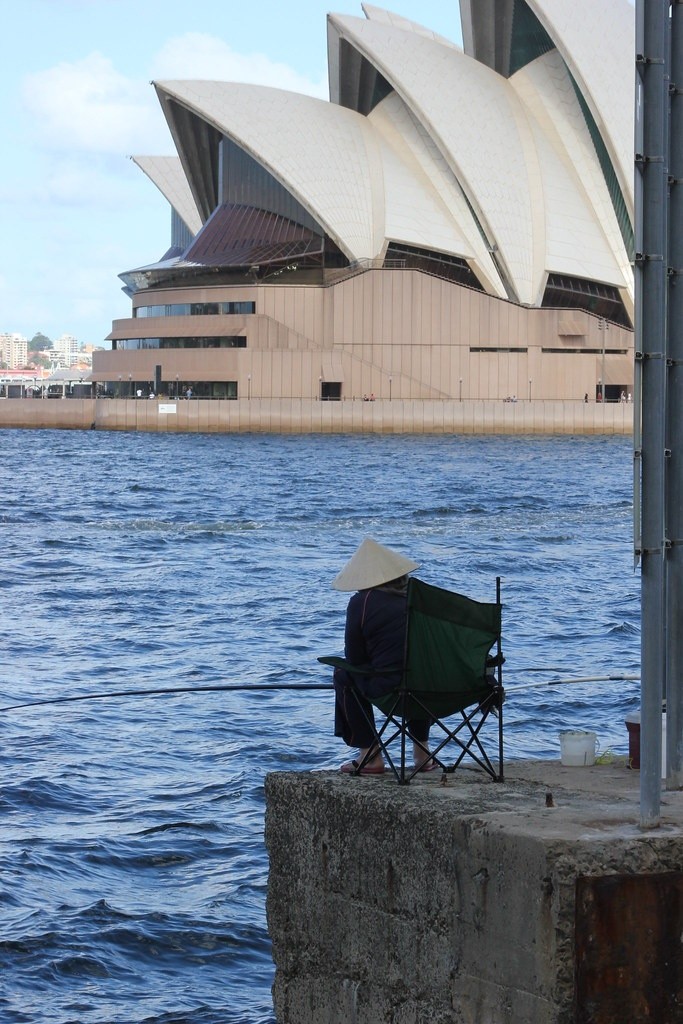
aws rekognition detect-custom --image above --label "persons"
[158,392,164,400]
[597,393,602,402]
[619,391,632,403]
[185,386,193,400]
[363,394,369,401]
[585,393,588,403]
[149,392,155,400]
[96,389,114,399]
[512,395,516,402]
[370,394,375,401]
[318,536,438,773]
[136,388,143,399]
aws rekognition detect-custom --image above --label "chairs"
[318,576,502,786]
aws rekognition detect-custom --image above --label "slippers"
[341,760,385,774]
[414,758,439,771]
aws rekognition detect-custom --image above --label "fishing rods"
[2,684,335,711]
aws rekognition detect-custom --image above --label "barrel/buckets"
[559,731,601,767]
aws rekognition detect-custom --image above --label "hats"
[332,538,421,591]
